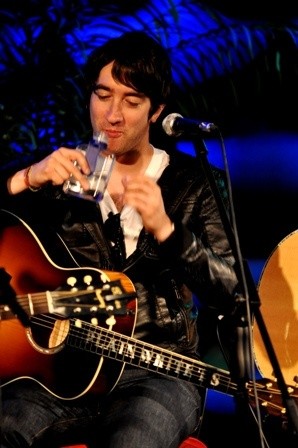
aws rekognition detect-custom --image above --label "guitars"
[0,267,140,330]
[0,201,298,431]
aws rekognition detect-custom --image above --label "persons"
[0,33,250,448]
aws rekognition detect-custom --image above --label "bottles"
[63,130,117,202]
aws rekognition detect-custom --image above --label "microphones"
[162,113,213,136]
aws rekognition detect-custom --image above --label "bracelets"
[22,163,43,194]
